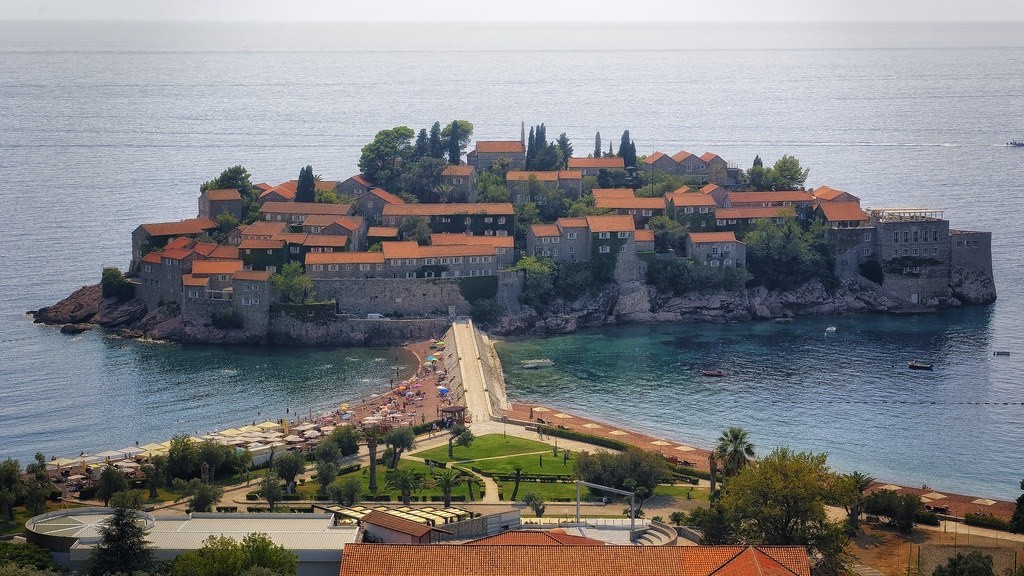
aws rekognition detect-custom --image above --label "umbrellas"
[47,413,320,486]
[299,339,446,428]
[530,404,696,462]
[878,482,996,514]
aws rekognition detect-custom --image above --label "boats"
[522,358,556,371]
[826,326,837,333]
[906,359,933,370]
[702,369,727,378]
[1006,138,1023,147]
[993,350,1012,356]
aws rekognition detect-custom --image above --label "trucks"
[367,313,389,319]
